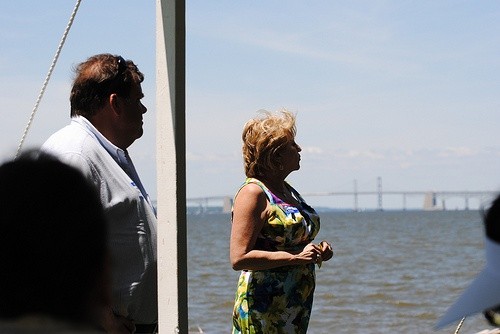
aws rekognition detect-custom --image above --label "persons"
[227,109,334,334]
[41,54,159,334]
[0,149,109,334]
[432,191,500,334]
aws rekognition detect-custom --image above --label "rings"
[317,254,320,259]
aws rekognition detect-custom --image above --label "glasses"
[100,55,126,106]
